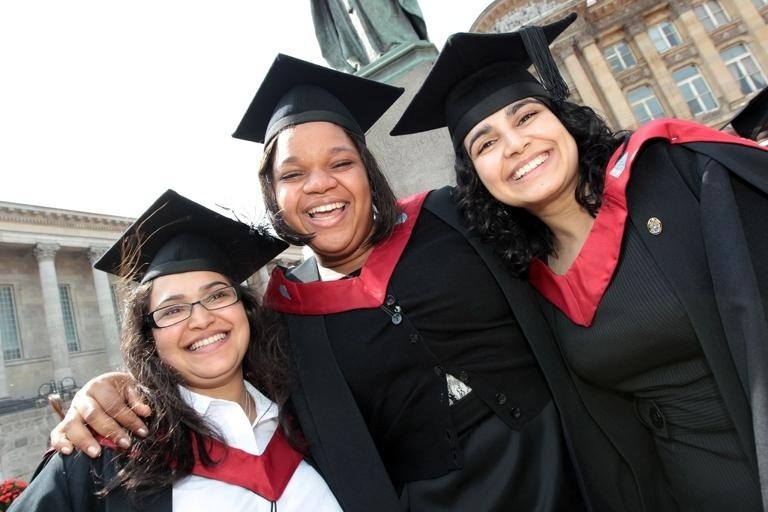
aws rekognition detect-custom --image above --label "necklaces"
[247,394,251,418]
[245,391,249,415]
[346,275,402,325]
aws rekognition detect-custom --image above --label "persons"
[725,83,767,145]
[393,10,767,512]
[48,48,642,512]
[3,186,352,512]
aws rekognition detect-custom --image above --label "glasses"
[136,285,247,329]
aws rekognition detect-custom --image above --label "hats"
[718,84,767,141]
[231,51,406,152]
[389,12,579,149]
[94,189,289,285]
